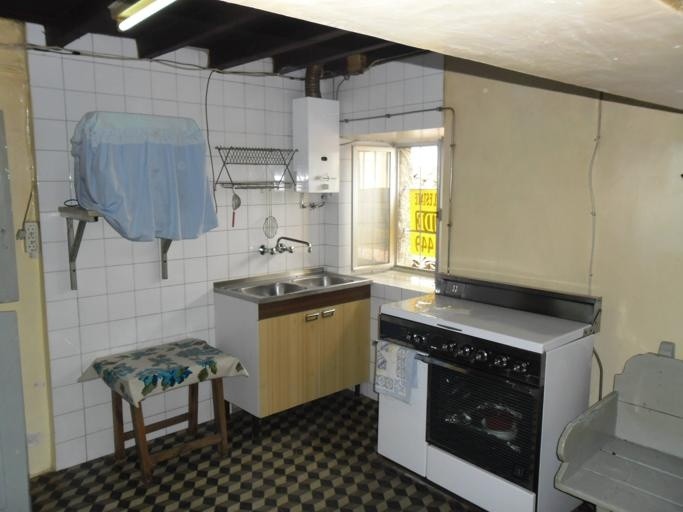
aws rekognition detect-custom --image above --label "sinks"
[242,282,306,297]
[292,272,356,287]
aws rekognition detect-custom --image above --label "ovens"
[378,314,546,512]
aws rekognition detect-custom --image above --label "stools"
[94,338,237,485]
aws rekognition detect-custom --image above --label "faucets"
[276,236,313,252]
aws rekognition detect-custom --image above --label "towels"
[374,340,418,404]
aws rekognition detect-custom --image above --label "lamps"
[107,0,176,31]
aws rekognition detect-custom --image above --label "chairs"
[554,341,683,512]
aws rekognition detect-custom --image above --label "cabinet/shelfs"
[214,284,370,446]
